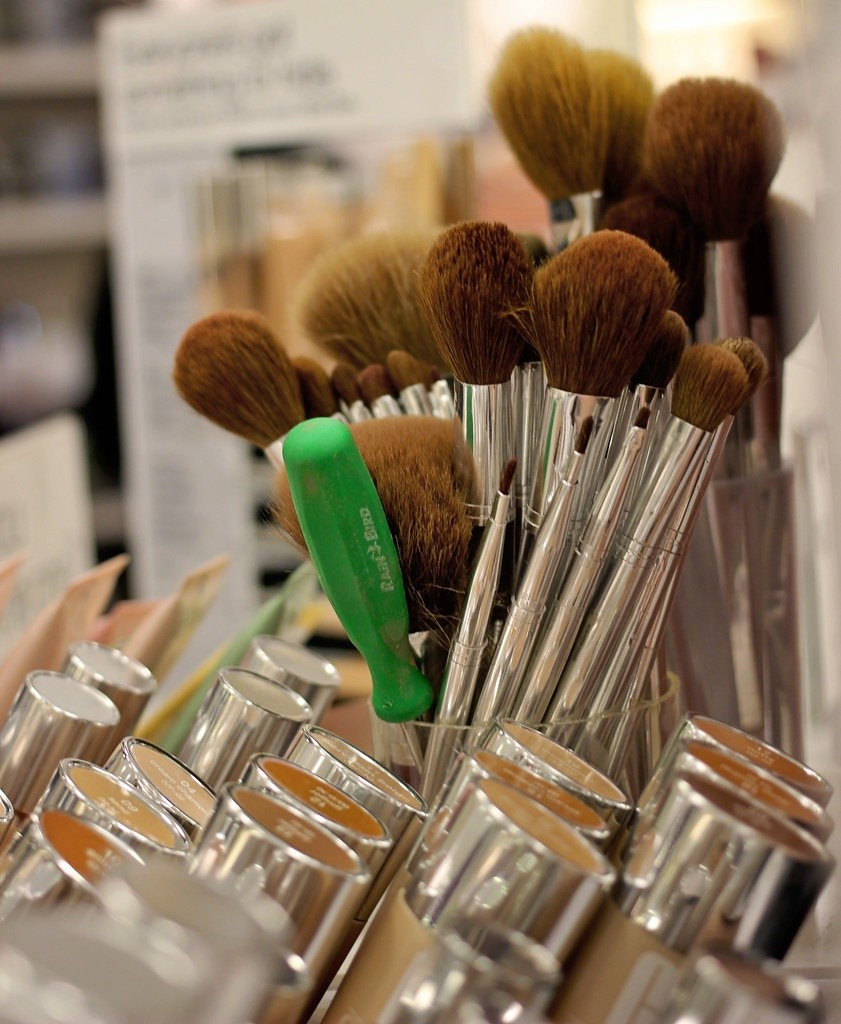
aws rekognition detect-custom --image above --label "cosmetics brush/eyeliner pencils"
[174,22,787,812]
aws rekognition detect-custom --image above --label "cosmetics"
[0,632,836,1024]
